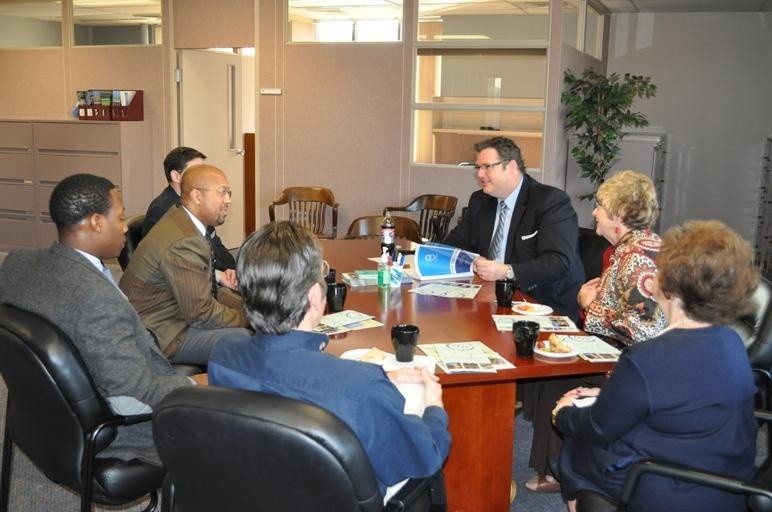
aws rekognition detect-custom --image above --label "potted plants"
[559,64,658,283]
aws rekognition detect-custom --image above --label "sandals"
[525,474,564,493]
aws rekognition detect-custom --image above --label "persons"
[144,147,239,290]
[0,172,208,470]
[551,217,760,508]
[118,166,256,368]
[526,171,673,491]
[432,137,585,325]
[206,220,452,504]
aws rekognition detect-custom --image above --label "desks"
[227,238,622,512]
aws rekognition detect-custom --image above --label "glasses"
[314,262,330,279]
[190,186,232,196]
[473,160,510,170]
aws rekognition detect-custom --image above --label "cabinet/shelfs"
[0,119,154,256]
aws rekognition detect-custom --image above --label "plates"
[534,341,580,357]
[512,301,553,315]
[340,348,394,366]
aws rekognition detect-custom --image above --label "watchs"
[505,264,514,281]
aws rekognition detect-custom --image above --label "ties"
[206,230,218,301]
[487,201,507,262]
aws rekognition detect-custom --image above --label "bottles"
[380,211,396,257]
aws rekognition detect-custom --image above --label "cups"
[497,280,513,307]
[393,326,420,361]
[327,284,346,311]
[326,269,336,286]
[514,322,540,357]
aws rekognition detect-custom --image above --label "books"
[313,319,383,335]
[490,315,580,333]
[354,269,380,279]
[86,90,101,105]
[404,243,479,281]
[77,90,83,105]
[408,282,482,300]
[434,344,497,377]
[557,335,622,362]
[110,90,136,119]
[99,91,112,105]
[341,273,414,288]
[418,341,517,375]
[319,308,375,329]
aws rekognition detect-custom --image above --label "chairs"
[724,276,772,365]
[150,385,448,512]
[382,194,458,245]
[268,186,340,240]
[561,410,772,512]
[0,301,171,510]
[342,215,423,243]
